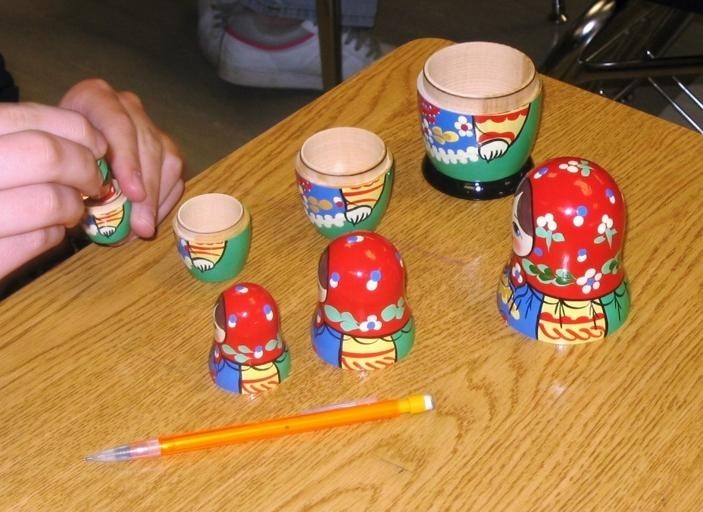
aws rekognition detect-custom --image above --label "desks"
[1,37,703,511]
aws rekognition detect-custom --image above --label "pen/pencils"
[82,394,435,463]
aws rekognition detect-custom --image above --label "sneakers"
[195,0,240,69]
[214,18,398,90]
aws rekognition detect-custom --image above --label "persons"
[0,51,185,302]
[197,0,397,90]
